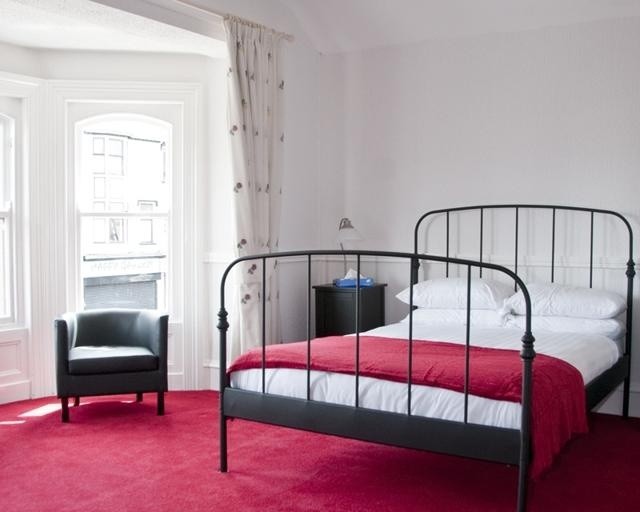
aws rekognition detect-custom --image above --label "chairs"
[56,309,170,422]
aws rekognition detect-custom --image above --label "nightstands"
[313,282,388,337]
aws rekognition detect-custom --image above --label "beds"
[216,206,636,511]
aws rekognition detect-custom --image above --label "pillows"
[395,277,627,339]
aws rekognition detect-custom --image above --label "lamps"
[335,215,357,283]
